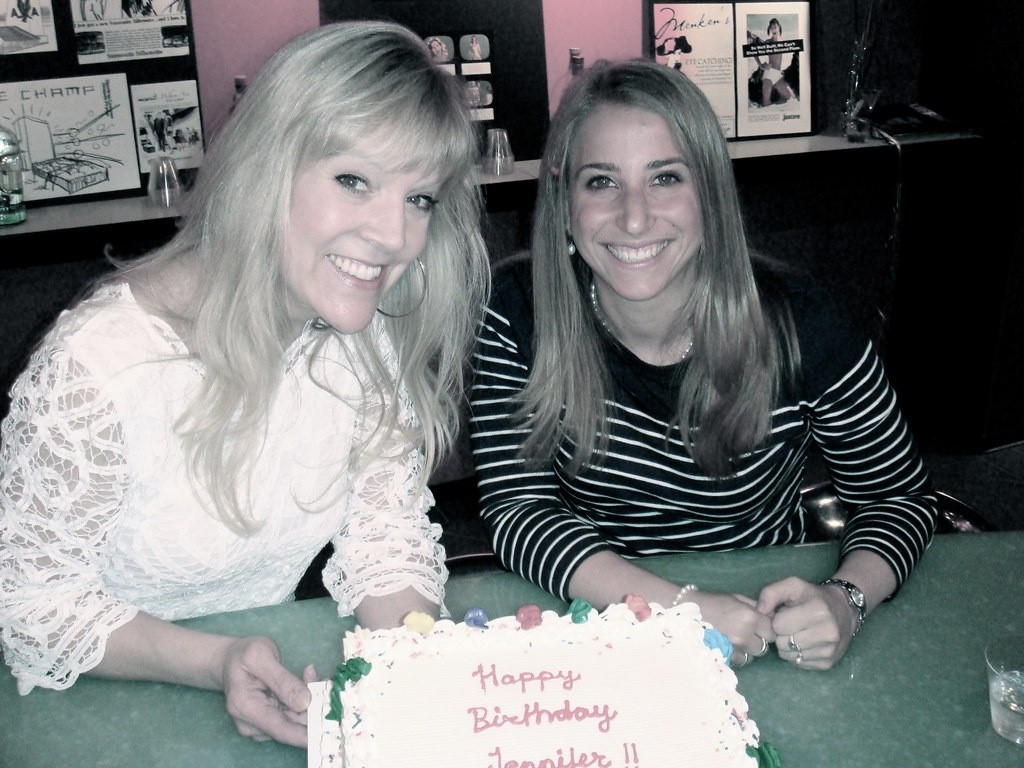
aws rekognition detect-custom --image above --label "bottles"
[228,75,249,112]
[563,48,584,82]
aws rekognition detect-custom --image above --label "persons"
[467,56,938,673]
[426,37,448,61]
[467,36,482,60]
[0,20,491,750]
[754,19,797,105]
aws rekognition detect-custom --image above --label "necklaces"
[591,280,694,362]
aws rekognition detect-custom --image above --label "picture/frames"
[648,0,820,144]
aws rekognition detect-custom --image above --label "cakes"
[320,594,784,768]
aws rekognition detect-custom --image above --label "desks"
[0,527,1024,768]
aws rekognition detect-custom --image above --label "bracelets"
[673,583,699,606]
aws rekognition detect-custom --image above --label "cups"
[481,127,515,175]
[147,156,185,209]
[984,633,1024,747]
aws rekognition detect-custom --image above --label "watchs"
[818,577,867,635]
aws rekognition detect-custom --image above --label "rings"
[752,638,769,657]
[790,635,797,650]
[797,645,801,652]
[795,653,804,665]
[734,652,749,667]
